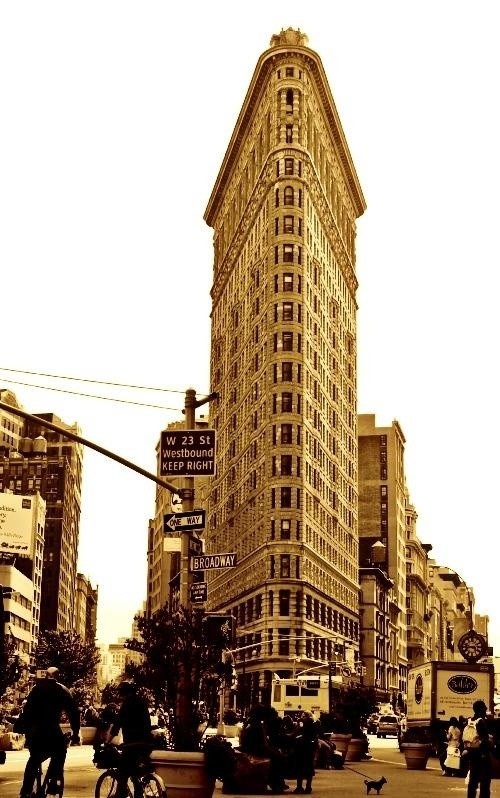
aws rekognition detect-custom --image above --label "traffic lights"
[334,643,338,655]
[340,644,344,654]
[357,667,361,675]
[362,666,367,676]
[208,616,235,642]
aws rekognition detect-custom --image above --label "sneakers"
[293,787,312,794]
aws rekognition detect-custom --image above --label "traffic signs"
[329,662,337,676]
[188,535,203,554]
[189,582,208,602]
[160,429,215,475]
[189,552,238,570]
[164,510,205,532]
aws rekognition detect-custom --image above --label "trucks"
[397,661,495,757]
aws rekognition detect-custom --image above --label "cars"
[365,711,402,739]
[147,706,175,726]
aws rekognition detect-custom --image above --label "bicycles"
[92,741,167,798]
[23,731,82,798]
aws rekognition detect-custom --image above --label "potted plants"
[399,724,433,769]
[29,629,98,743]
[319,682,379,763]
[101,605,235,798]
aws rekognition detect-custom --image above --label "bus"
[271,674,356,727]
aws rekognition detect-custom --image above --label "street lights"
[428,563,473,631]
[292,658,301,680]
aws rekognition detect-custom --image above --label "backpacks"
[462,717,484,749]
[441,745,462,770]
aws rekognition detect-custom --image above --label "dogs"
[364,776,387,795]
